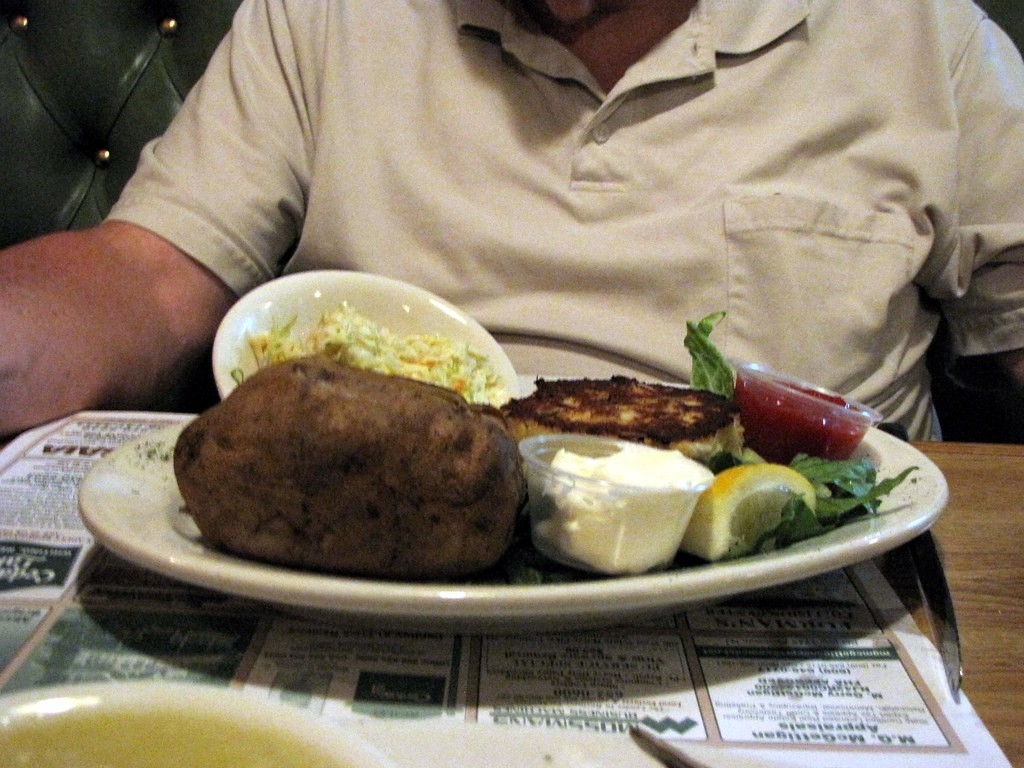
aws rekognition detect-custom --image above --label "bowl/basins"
[728,358,885,466]
[211,271,519,409]
[0,682,398,768]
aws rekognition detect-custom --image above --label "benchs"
[0,0,1024,444]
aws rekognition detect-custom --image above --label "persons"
[0,0,1024,438]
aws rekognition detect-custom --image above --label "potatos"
[173,357,525,582]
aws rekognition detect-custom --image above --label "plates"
[78,424,949,633]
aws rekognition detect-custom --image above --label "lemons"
[683,462,816,562]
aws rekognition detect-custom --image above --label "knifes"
[910,530,964,703]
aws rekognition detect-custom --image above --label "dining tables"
[0,409,1024,768]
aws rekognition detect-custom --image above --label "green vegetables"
[683,313,921,548]
